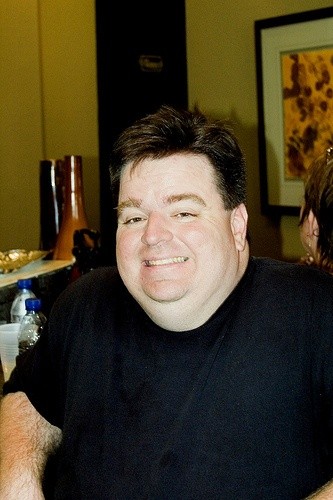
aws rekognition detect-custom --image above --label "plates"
[0,248,49,274]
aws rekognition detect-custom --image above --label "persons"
[297,146,333,274]
[0,106,333,500]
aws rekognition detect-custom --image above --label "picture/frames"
[254,6,333,216]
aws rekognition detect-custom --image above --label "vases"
[50,155,92,285]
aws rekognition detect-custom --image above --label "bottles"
[18,298,47,355]
[11,279,36,322]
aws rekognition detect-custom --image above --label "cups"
[0,323,22,383]
